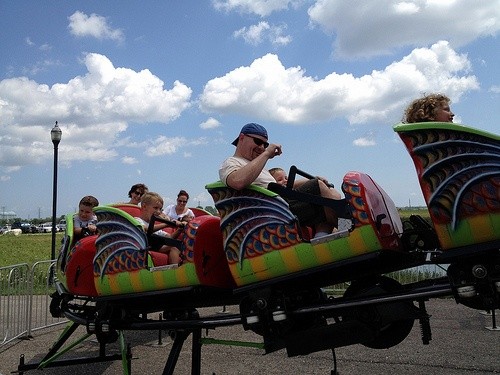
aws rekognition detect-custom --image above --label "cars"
[0,220,67,234]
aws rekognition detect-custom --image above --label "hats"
[230,123,268,147]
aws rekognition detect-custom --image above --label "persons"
[163,189,195,219]
[133,192,187,266]
[219,122,350,237]
[74,196,99,235]
[178,215,195,223]
[269,167,341,196]
[404,93,455,122]
[129,184,149,204]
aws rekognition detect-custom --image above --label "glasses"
[243,134,269,148]
[133,191,144,197]
[177,199,187,203]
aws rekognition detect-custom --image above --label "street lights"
[49,121,62,289]
